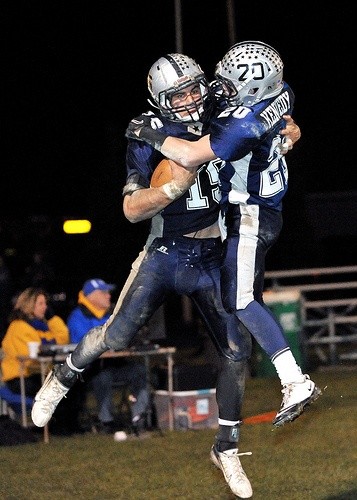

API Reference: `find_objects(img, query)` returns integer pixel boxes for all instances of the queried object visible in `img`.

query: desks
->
[16,347,176,444]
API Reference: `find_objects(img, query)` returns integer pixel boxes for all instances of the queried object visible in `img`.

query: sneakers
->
[31,364,70,427]
[273,374,323,428]
[210,444,253,498]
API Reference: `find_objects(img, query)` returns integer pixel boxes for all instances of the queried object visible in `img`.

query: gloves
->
[126,111,169,151]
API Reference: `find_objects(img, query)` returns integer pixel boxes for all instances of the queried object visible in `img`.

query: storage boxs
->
[154,388,219,432]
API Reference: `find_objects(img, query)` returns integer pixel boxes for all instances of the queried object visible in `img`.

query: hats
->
[83,279,115,297]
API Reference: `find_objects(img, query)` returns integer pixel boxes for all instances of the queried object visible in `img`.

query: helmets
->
[209,41,284,107]
[147,54,209,123]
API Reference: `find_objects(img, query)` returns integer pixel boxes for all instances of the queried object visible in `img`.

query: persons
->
[31,53,301,499]
[125,40,323,430]
[1,277,156,434]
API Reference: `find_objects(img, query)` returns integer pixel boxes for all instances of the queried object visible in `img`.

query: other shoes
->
[103,420,143,436]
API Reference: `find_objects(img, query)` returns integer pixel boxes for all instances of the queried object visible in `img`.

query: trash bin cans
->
[247,290,304,379]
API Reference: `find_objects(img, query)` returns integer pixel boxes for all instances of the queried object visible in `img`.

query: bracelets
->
[162,181,182,199]
